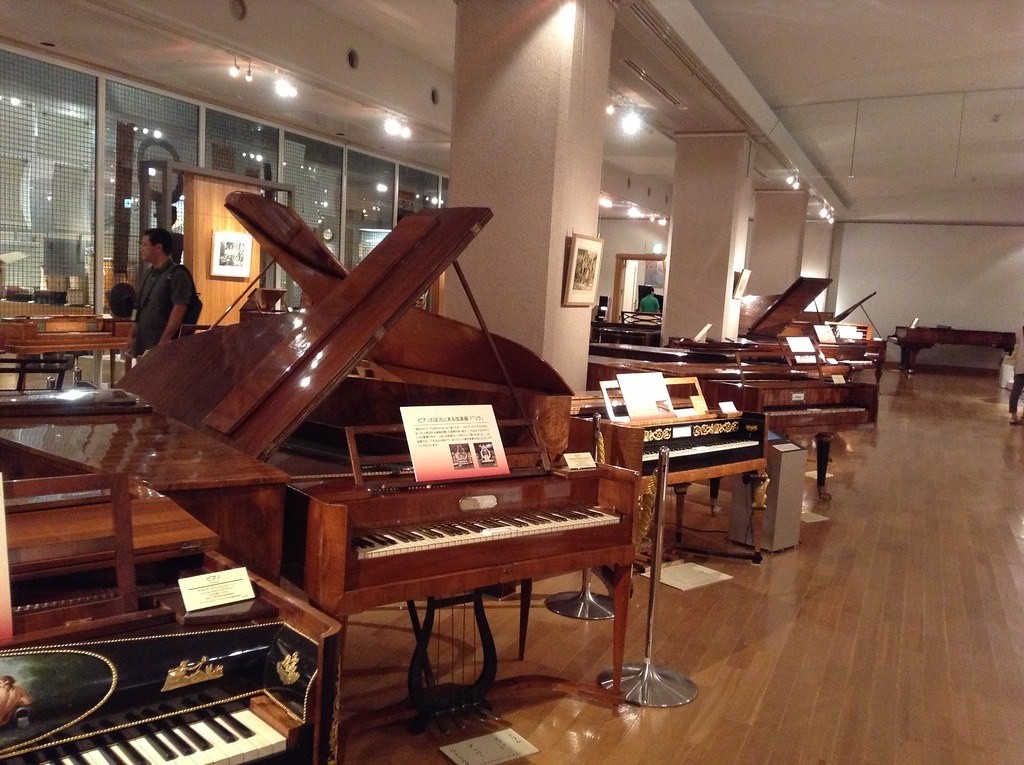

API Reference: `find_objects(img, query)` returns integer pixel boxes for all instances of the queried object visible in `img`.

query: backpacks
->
[170,265,203,339]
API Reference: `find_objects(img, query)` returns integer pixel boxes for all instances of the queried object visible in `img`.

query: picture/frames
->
[733,269,752,301]
[562,233,603,307]
[209,229,252,278]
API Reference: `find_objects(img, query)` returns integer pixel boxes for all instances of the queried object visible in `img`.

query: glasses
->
[141,242,152,247]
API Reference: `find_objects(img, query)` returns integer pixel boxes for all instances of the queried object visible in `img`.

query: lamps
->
[229,58,241,78]
[245,63,253,82]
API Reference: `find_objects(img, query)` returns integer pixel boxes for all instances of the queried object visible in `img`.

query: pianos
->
[746,276,889,383]
[698,350,880,518]
[1,469,346,765]
[565,370,771,566]
[888,316,1016,375]
[108,185,649,739]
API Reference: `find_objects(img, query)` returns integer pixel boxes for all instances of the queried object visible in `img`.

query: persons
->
[639,287,660,313]
[1008,325,1024,424]
[125,228,194,361]
